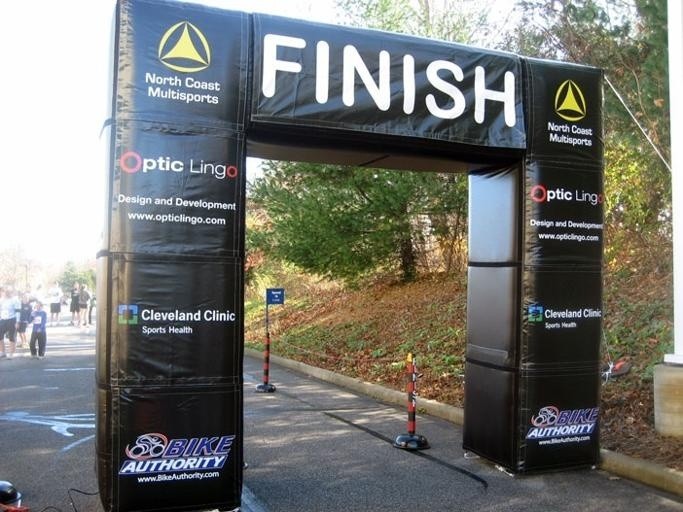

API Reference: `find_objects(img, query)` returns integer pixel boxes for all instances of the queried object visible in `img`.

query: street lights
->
[20,264,28,290]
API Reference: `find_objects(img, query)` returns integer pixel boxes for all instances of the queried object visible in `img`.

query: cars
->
[61,296,71,304]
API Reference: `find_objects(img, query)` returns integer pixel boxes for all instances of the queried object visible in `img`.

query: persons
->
[0,278,91,360]
